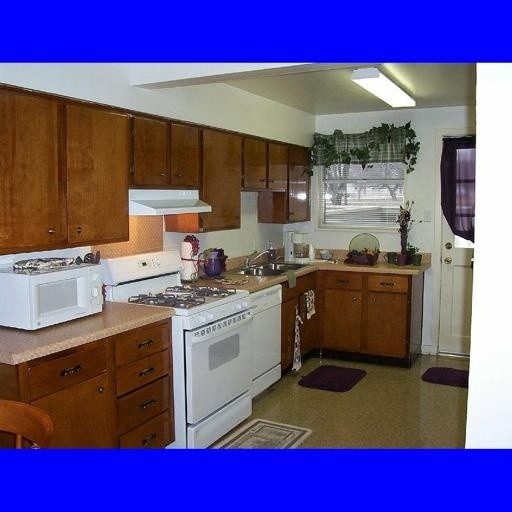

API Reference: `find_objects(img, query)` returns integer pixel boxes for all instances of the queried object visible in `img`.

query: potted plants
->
[343,246,381,266]
[395,199,422,267]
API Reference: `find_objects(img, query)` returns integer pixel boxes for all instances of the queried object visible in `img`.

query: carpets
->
[421,367,468,389]
[299,365,366,393]
[212,419,314,450]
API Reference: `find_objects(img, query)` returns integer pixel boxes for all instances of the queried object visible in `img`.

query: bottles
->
[267,239,277,262]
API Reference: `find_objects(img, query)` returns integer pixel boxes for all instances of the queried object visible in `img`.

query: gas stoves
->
[127,283,251,329]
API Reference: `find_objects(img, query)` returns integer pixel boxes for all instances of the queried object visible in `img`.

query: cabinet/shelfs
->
[165,129,240,233]
[241,137,287,191]
[256,145,310,222]
[116,321,175,450]
[0,337,117,450]
[319,270,424,363]
[0,85,129,254]
[282,271,318,371]
[130,115,200,191]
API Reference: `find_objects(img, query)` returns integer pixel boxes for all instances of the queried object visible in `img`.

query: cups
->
[394,253,407,266]
[409,254,423,266]
[383,251,398,264]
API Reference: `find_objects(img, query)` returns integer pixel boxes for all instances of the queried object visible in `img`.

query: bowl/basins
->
[320,249,334,259]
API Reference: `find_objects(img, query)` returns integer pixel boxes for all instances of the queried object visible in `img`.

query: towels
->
[291,305,303,371]
[303,290,317,321]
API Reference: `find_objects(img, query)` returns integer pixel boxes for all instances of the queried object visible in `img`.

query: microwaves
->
[0,263,104,332]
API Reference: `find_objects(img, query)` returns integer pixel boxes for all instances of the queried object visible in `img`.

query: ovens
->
[174,307,253,448]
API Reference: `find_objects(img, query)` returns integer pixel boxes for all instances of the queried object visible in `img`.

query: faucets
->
[243,250,270,267]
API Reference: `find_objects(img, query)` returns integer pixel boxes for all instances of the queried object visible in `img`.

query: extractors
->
[127,188,212,216]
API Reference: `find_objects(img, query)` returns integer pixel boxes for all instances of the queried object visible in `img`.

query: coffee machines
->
[283,230,316,261]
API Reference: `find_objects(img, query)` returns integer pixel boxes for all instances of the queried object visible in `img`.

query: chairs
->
[0,399,54,449]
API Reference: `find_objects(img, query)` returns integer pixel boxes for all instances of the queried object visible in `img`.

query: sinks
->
[234,262,305,277]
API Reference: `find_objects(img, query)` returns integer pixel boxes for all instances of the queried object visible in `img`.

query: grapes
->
[344,250,357,264]
[208,248,228,272]
[185,235,199,255]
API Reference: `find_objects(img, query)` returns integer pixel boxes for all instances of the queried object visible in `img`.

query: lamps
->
[351,68,417,108]
